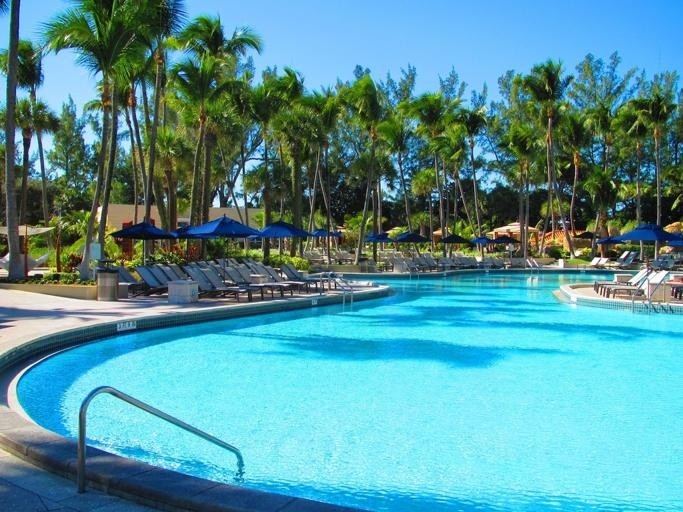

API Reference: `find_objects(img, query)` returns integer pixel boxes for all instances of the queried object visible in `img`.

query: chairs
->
[594,267,670,302]
[378,249,509,274]
[649,252,683,270]
[587,249,638,268]
[136,256,340,304]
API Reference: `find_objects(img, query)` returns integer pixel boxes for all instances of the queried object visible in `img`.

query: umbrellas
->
[170,222,222,265]
[437,234,471,256]
[309,227,341,255]
[362,232,395,260]
[183,213,259,263]
[245,218,314,258]
[469,235,497,258]
[392,230,430,257]
[107,216,178,267]
[570,222,683,269]
[491,234,521,251]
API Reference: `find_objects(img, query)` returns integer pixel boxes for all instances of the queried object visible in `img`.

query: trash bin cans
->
[95,258,120,301]
